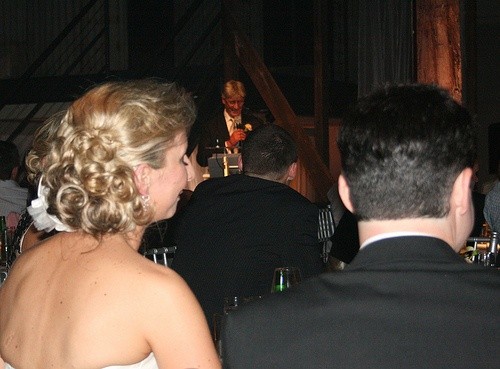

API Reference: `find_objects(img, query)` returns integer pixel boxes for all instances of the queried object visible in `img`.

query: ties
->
[229,119,237,137]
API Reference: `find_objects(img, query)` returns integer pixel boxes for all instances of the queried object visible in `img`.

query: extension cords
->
[223,155,229,177]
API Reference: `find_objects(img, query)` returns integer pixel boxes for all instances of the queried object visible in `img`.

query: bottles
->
[486,232,500,268]
[0,216,11,267]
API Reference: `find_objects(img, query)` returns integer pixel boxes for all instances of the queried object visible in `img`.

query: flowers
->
[243,123,253,132]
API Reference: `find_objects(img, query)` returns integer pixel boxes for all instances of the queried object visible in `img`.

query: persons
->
[172,124,320,343]
[196,78,265,167]
[0,78,222,369]
[220,82,500,369]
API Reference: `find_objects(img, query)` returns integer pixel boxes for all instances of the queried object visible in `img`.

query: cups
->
[223,296,240,316]
[270,266,303,295]
[2,225,15,268]
